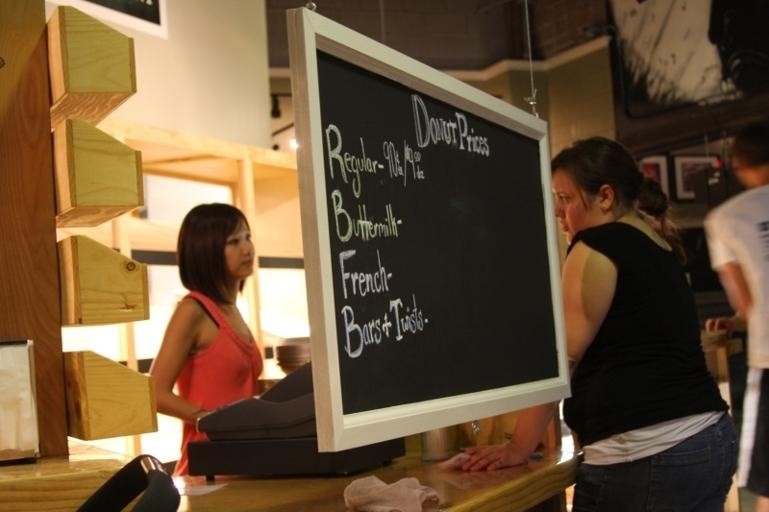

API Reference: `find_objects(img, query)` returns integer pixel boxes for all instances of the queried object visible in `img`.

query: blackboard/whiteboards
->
[285,7,574,455]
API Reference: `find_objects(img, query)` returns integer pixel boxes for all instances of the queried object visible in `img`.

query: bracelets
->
[185,409,209,422]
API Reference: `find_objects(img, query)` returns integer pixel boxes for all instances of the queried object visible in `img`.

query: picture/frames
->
[672,154,721,203]
[639,153,673,203]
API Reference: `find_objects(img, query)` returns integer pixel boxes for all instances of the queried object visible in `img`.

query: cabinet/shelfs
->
[46,5,159,441]
[109,139,311,459]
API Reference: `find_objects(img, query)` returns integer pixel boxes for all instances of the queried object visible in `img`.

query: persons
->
[457,134,742,510]
[702,122,768,511]
[147,200,273,483]
